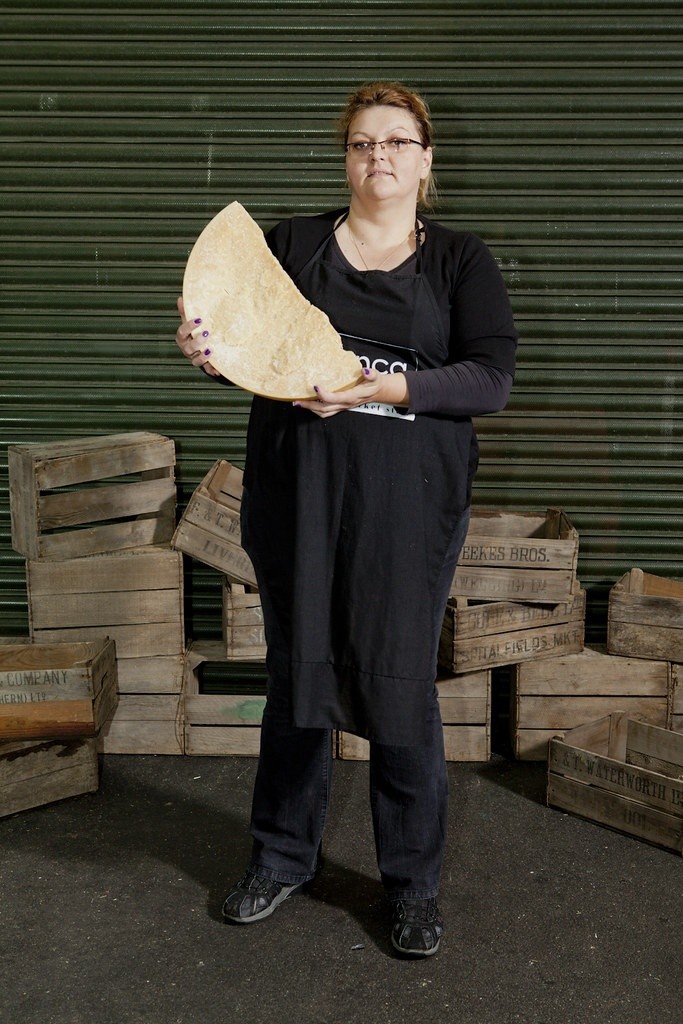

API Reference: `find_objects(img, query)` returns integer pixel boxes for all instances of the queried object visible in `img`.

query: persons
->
[175,79,521,957]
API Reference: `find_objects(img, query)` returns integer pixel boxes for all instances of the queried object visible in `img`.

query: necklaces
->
[348,219,414,269]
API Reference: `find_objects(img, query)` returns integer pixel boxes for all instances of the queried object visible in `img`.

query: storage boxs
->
[1,429,682,854]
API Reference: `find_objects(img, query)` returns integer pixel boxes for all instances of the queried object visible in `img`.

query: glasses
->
[345,137,425,157]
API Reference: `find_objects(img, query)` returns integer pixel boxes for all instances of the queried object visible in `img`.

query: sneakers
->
[391,892,444,954]
[222,871,303,923]
